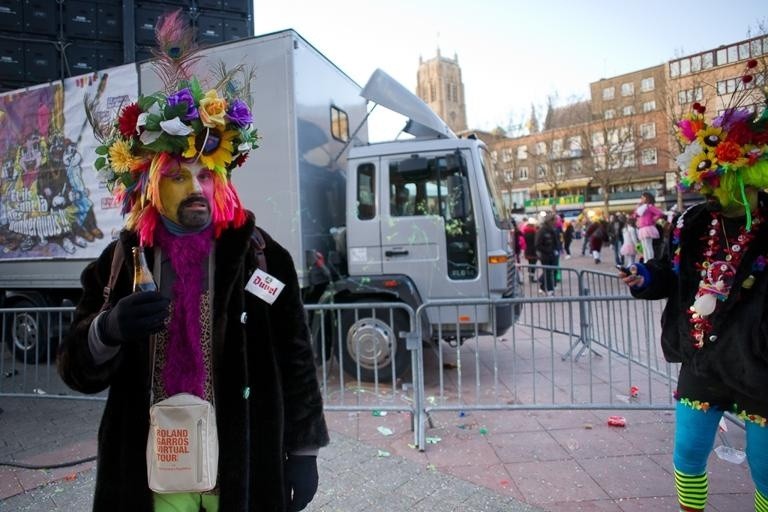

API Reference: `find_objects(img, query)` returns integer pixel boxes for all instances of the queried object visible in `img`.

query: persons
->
[617,176,768,512]
[54,160,330,512]
[514,190,677,297]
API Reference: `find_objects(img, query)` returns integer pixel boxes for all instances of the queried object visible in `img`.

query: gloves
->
[284,453,319,512]
[95,289,171,345]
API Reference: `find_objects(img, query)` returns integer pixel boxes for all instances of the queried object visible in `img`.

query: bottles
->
[132,247,164,335]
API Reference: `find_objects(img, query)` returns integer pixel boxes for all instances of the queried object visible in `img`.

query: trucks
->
[0,29,524,384]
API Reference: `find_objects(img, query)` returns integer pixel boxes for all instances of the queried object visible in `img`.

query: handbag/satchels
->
[144,390,219,493]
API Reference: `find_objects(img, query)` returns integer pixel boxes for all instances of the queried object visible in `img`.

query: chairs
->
[395,186,410,216]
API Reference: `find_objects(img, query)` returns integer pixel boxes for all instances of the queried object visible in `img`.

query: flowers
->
[92,73,262,191]
[675,58,767,190]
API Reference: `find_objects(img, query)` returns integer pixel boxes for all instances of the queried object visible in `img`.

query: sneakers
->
[537,288,555,297]
